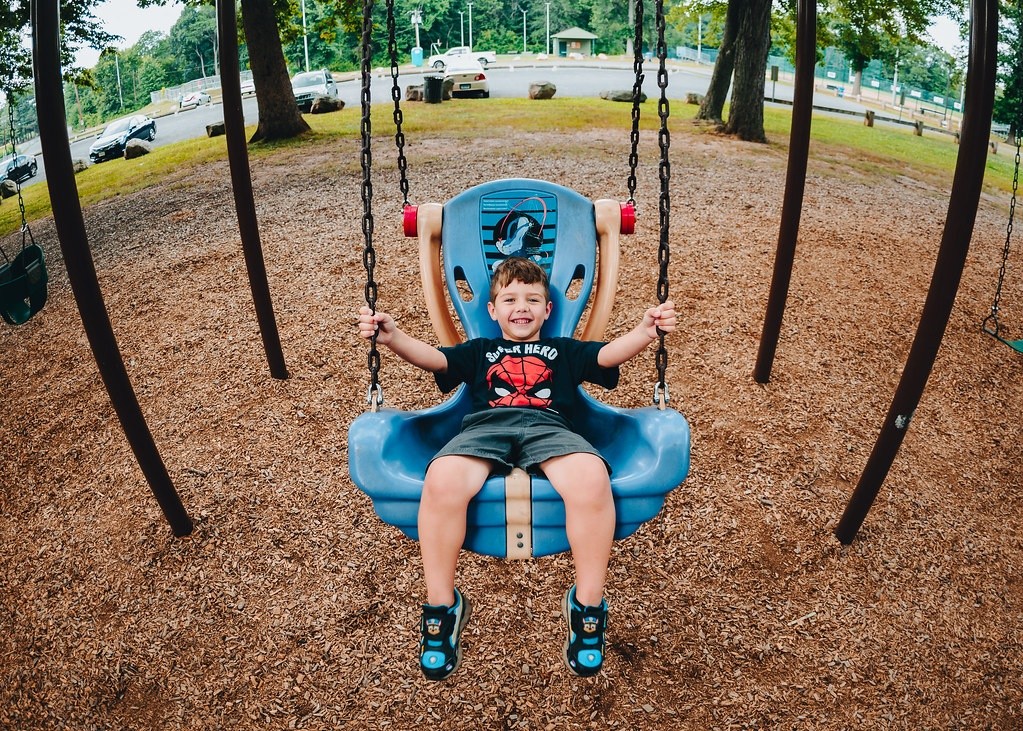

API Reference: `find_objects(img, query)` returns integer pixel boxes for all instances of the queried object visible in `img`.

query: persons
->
[358,257,676,682]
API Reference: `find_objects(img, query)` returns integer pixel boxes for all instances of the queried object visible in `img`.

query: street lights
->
[544,3,551,55]
[457,11,466,47]
[521,10,529,54]
[467,2,474,52]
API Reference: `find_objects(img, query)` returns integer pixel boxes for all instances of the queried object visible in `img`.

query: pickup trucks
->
[428,46,496,70]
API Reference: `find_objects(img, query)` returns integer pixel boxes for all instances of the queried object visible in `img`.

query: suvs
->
[290,68,338,114]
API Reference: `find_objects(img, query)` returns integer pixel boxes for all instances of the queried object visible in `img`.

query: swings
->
[981,77,1023,356]
[1,0,51,326]
[348,2,692,562]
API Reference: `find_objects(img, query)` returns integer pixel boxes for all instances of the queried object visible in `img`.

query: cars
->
[240,79,256,96]
[89,115,157,164]
[0,155,38,195]
[443,60,489,99]
[182,92,211,107]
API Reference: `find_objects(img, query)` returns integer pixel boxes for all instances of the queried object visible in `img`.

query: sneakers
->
[419,586,472,682]
[560,584,607,678]
